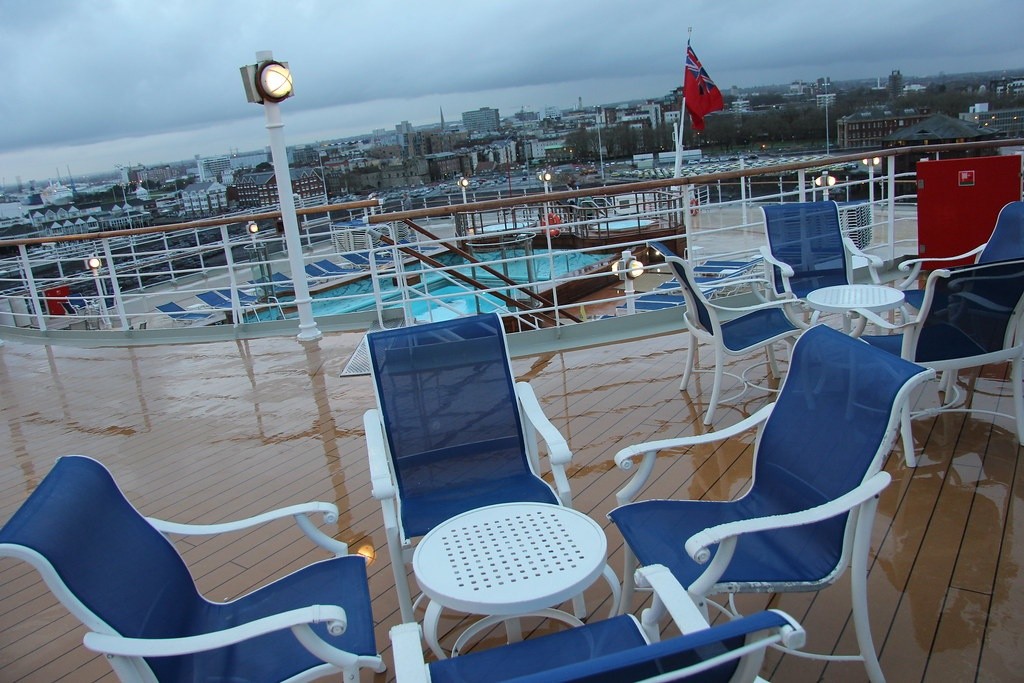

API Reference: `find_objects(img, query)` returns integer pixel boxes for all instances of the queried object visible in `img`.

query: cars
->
[610,163,740,179]
[688,154,757,164]
[378,187,432,198]
[440,176,506,193]
[745,156,856,176]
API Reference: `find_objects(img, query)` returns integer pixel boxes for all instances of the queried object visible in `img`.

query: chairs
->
[388,564,808,683]
[847,256,1024,467]
[646,240,805,424]
[893,201,1024,391]
[0,455,387,683]
[58,287,123,330]
[612,322,935,683]
[154,273,318,328]
[782,199,872,257]
[304,240,439,283]
[593,257,764,322]
[759,200,884,367]
[362,312,586,623]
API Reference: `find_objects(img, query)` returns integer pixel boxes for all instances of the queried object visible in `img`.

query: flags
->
[683,41,723,130]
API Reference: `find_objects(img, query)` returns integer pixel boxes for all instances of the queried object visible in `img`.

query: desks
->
[807,284,910,327]
[413,501,623,662]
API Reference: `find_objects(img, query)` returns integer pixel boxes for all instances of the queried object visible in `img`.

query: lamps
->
[256,60,293,103]
[612,262,619,276]
[460,179,469,187]
[626,259,643,278]
[88,258,100,268]
[544,173,551,181]
[249,223,259,233]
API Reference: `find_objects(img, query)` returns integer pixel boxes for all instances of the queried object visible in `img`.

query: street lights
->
[863,156,880,223]
[539,170,552,213]
[815,171,836,201]
[240,52,323,343]
[611,250,644,315]
[245,220,270,303]
[457,177,470,229]
[84,254,112,329]
